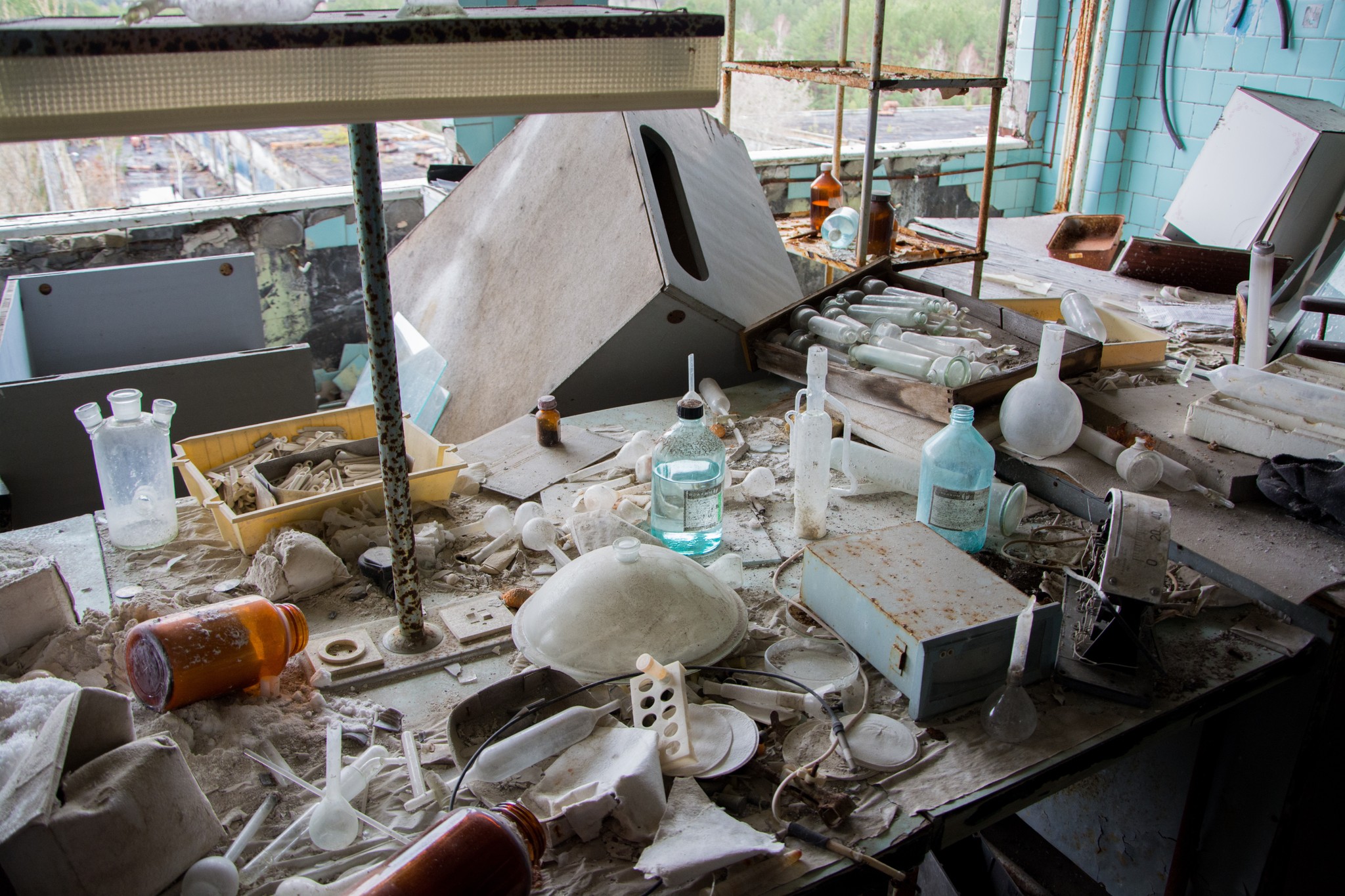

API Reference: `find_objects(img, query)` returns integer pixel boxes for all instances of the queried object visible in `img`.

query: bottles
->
[75,387,177,550]
[811,160,844,230]
[867,189,897,258]
[821,206,859,248]
[916,404,995,554]
[536,396,562,446]
[998,323,1083,456]
[650,397,725,555]
[126,594,309,713]
[1060,290,1108,344]
[348,801,546,896]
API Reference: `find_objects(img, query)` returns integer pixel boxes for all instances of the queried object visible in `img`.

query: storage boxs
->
[172,402,469,555]
[743,255,1103,424]
[1047,215,1125,271]
[982,296,1169,366]
[800,522,1062,720]
[1184,353,1345,460]
[250,437,415,510]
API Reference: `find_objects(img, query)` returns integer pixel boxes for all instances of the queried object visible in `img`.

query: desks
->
[1,212,1345,896]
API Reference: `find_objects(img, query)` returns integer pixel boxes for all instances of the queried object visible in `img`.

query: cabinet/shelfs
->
[1152,86,1345,304]
[386,109,803,444]
[724,1,1012,298]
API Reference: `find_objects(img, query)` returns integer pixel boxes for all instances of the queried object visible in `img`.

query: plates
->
[785,591,837,639]
[764,637,861,693]
